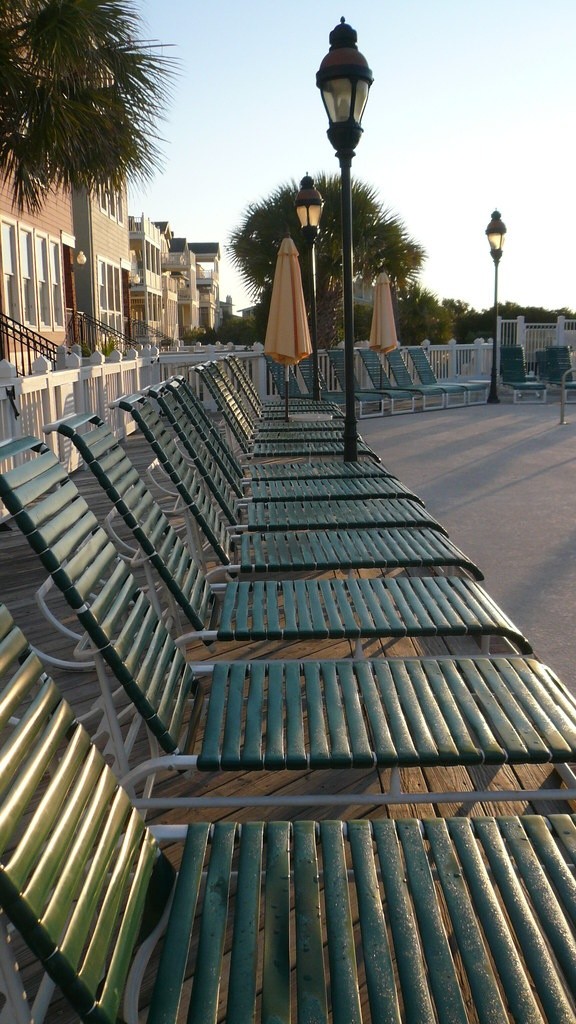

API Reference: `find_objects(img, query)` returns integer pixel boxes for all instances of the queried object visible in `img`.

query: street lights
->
[484,208,507,403]
[296,170,328,402]
[315,18,372,464]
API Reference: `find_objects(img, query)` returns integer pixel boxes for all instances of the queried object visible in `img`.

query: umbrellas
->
[368,273,397,410]
[265,230,313,421]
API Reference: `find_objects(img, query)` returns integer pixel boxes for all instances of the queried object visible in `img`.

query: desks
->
[288,413,332,421]
[468,379,491,384]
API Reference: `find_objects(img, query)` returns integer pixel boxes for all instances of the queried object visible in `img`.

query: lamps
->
[76,251,87,267]
[128,274,140,288]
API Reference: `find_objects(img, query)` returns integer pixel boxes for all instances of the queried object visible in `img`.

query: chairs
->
[0,345,576,1024]
